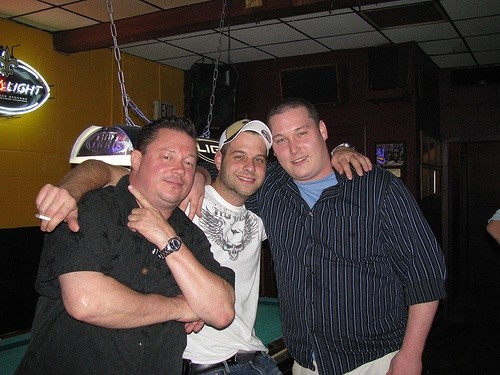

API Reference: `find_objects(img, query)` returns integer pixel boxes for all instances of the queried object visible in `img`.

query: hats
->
[219,119,273,156]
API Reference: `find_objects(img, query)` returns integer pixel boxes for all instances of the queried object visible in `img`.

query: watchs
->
[156,236,182,259]
[331,142,355,156]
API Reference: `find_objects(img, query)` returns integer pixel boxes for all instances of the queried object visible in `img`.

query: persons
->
[14,116,235,375]
[179,99,447,374]
[36,117,372,375]
[486,209,500,242]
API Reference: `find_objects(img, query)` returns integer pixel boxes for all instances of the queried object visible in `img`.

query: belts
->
[191,351,261,374]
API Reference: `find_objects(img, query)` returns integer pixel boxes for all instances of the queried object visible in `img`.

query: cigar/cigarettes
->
[35,213,52,221]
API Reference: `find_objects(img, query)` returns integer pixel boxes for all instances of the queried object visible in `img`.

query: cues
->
[271,348,289,360]
